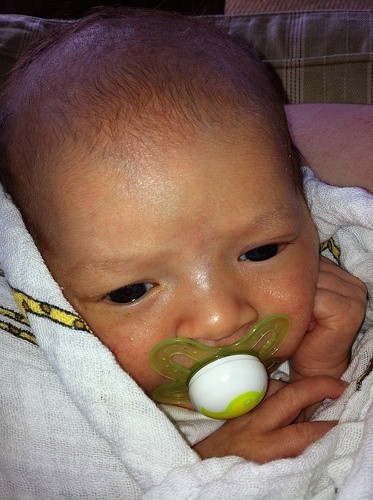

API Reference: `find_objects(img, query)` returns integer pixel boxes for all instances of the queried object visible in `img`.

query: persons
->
[1,10,373,500]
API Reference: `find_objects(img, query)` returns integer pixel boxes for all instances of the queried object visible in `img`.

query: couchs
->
[0,10,373,195]
